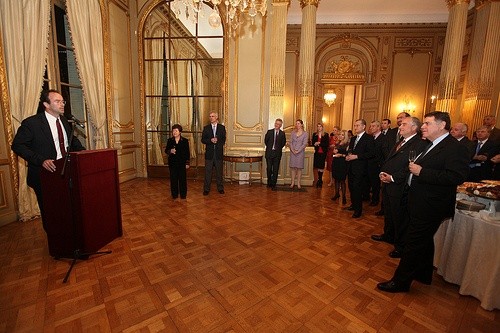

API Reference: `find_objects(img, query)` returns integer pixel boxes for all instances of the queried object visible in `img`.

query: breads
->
[456,180,500,212]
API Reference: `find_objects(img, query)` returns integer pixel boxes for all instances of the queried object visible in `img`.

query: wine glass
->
[408,150,416,174]
[488,200,496,217]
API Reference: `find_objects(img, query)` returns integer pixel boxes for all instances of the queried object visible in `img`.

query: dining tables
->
[432,186,500,312]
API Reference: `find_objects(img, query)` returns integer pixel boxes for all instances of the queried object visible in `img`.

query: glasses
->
[53,100,66,105]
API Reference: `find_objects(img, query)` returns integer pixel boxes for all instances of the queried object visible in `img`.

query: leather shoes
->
[389,248,402,258]
[375,210,384,217]
[411,274,433,284]
[344,205,363,218]
[362,196,380,206]
[377,279,410,294]
[371,234,392,245]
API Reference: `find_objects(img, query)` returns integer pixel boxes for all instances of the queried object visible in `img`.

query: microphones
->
[68,114,85,129]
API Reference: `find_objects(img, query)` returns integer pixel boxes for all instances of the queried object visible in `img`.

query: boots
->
[316,172,324,187]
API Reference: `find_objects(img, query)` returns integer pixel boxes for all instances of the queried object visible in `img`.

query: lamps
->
[324,89,337,107]
[172,0,268,38]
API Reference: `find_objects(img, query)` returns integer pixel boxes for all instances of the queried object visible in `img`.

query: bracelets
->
[342,154,343,157]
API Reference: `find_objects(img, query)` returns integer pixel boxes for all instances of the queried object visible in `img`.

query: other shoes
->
[275,131,278,147]
[171,194,186,200]
[203,190,210,196]
[219,190,225,194]
[267,182,276,188]
[213,126,216,139]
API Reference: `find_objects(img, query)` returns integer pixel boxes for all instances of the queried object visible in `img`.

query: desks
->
[223,155,264,186]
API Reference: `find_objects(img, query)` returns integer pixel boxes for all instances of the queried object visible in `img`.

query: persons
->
[289,119,307,191]
[10,89,87,256]
[450,115,500,185]
[264,118,286,191]
[201,110,226,196]
[345,111,459,293]
[312,122,354,205]
[165,124,190,199]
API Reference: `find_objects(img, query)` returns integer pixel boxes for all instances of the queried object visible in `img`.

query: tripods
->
[54,122,112,283]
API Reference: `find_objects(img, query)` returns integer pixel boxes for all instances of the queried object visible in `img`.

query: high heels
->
[331,194,347,205]
[289,182,303,190]
[327,181,335,186]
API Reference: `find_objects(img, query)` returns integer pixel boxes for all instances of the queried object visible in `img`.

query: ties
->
[383,131,385,135]
[373,135,376,139]
[475,142,482,155]
[396,130,402,143]
[55,119,69,158]
[353,135,359,150]
[407,142,433,178]
[395,140,406,153]
